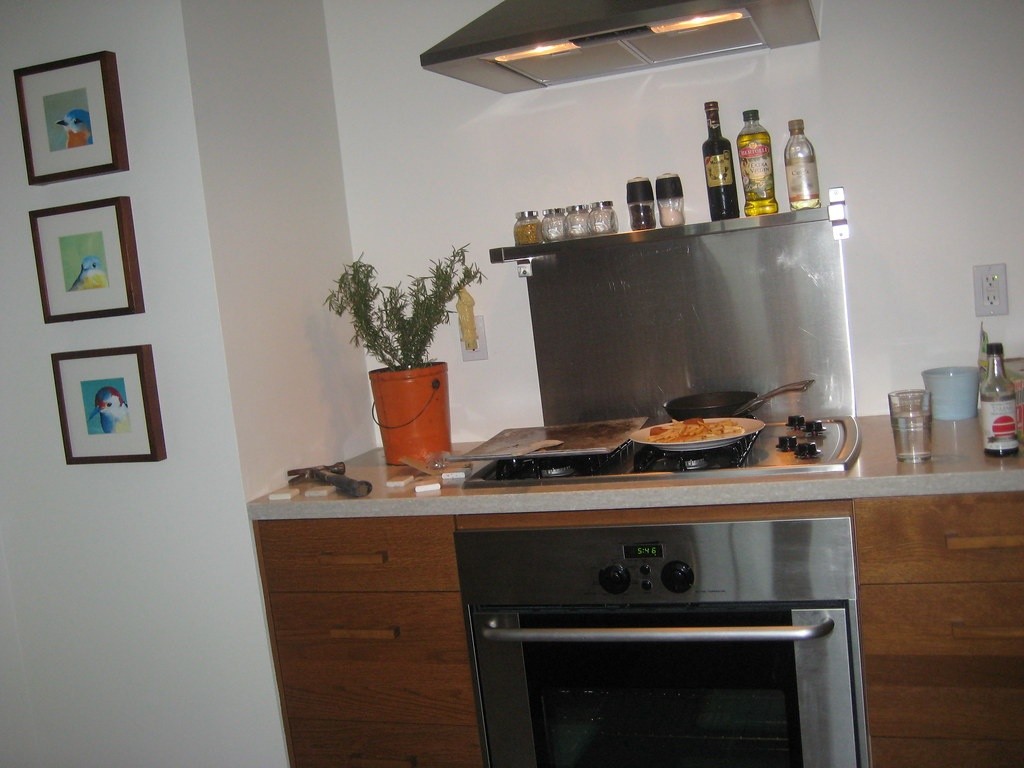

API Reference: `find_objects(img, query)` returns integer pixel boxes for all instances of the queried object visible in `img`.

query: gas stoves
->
[464,417,856,486]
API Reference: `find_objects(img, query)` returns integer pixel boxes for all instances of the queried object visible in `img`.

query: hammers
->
[287,461,373,497]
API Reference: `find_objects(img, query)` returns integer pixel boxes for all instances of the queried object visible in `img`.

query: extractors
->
[419,0,821,95]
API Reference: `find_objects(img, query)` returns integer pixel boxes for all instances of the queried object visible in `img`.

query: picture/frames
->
[51,344,167,464]
[28,196,146,323]
[13,51,131,186]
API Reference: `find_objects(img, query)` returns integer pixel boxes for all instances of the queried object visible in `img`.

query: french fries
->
[645,418,744,443]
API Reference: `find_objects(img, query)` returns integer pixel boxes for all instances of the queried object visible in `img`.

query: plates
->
[628,418,766,450]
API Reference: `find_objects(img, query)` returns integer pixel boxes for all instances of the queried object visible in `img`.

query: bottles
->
[512,199,619,246]
[655,173,685,228]
[626,178,657,231]
[980,342,1021,458]
[784,118,822,211]
[737,108,780,217]
[702,101,741,221]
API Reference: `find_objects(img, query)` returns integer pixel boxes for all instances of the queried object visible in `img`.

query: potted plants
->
[323,242,488,467]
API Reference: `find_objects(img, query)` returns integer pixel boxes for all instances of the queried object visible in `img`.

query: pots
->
[662,379,815,419]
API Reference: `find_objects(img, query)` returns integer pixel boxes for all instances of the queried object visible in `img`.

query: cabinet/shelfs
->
[253,515,488,768]
[854,490,1024,768]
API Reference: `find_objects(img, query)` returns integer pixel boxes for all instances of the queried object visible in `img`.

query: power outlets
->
[973,263,1009,317]
[459,315,488,362]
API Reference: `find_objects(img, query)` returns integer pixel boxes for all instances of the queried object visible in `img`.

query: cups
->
[888,389,932,465]
[921,367,979,421]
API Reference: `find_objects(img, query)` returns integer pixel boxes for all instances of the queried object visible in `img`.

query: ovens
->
[449,516,873,768]
